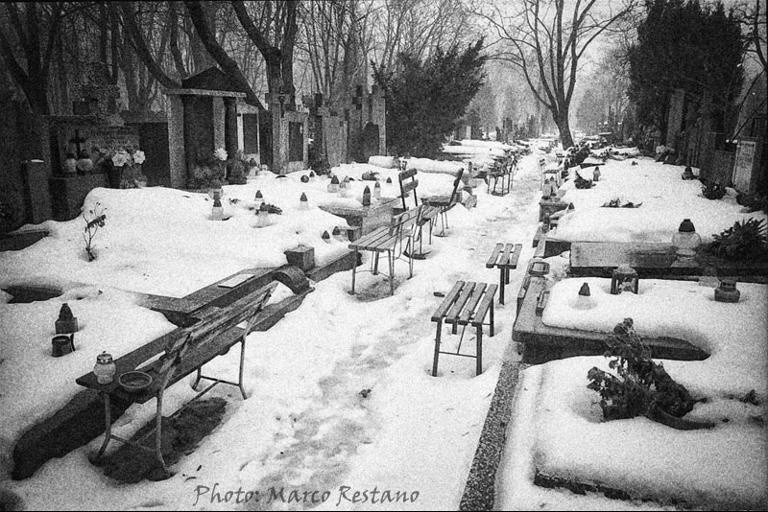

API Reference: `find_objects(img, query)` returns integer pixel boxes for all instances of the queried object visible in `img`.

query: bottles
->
[94,354,116,385]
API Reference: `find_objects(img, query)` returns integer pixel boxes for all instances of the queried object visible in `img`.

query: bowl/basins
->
[119,371,153,393]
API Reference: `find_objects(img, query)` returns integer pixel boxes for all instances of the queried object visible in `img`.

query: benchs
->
[78,156,569,481]
